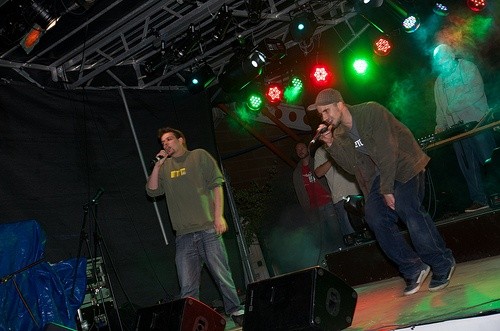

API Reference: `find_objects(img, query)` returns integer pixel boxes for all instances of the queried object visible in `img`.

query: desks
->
[424,121,500,150]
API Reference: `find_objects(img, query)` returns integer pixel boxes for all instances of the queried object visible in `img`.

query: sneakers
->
[403,266,431,296]
[464,203,489,213]
[428,258,456,293]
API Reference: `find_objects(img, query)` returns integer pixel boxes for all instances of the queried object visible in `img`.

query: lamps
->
[27,0,62,32]
[145,0,487,113]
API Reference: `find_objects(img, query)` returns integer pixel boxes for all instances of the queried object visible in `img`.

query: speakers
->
[242,265,358,331]
[132,297,226,331]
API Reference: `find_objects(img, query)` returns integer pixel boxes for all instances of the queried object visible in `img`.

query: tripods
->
[89,204,137,331]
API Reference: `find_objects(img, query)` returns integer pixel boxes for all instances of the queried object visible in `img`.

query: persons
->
[293,142,346,268]
[314,142,365,242]
[146,128,245,325]
[307,88,456,296]
[433,44,500,212]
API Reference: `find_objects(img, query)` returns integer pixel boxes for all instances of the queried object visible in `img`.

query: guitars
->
[418,115,479,148]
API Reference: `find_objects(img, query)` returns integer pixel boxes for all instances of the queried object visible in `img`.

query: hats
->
[307,88,344,111]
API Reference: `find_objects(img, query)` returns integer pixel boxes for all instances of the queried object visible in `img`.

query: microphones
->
[91,187,104,204]
[311,119,332,143]
[151,148,169,165]
